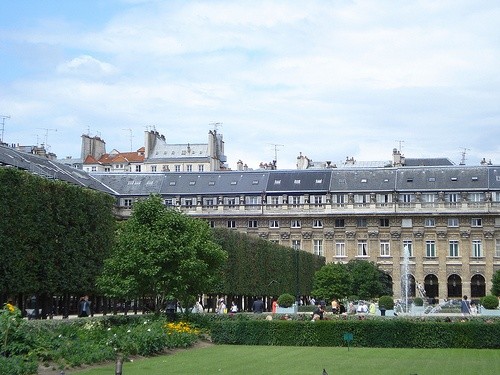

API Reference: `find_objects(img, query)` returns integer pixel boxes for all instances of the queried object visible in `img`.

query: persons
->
[369,300,375,314]
[320,298,326,311]
[310,301,323,322]
[192,302,203,312]
[461,295,472,315]
[300,299,303,306]
[310,297,316,306]
[79,295,91,317]
[331,298,337,314]
[348,302,356,315]
[218,301,226,314]
[358,302,368,313]
[339,302,345,314]
[432,298,436,308]
[230,300,239,313]
[252,296,265,314]
[271,299,279,314]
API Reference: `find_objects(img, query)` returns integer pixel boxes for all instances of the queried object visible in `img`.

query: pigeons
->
[322,368,328,375]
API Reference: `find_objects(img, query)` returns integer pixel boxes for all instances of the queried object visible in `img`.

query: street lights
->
[293,243,302,306]
